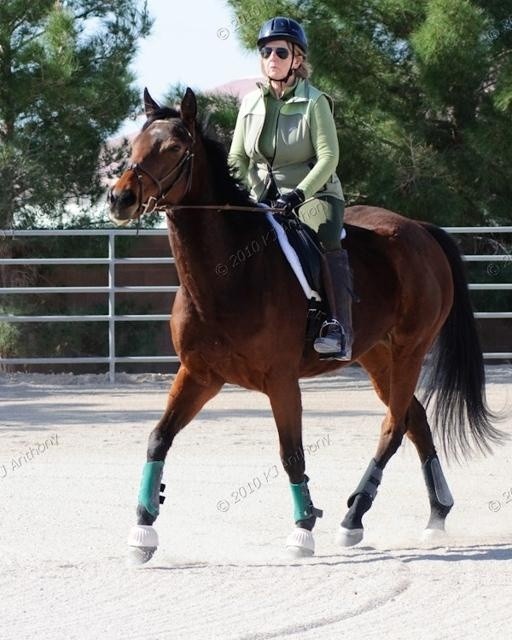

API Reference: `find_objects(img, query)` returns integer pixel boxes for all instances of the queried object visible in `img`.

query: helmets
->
[256,16,307,53]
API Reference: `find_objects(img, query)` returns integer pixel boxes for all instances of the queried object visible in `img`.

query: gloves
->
[274,189,305,215]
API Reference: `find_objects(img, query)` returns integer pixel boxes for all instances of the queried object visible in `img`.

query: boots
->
[314,250,354,362]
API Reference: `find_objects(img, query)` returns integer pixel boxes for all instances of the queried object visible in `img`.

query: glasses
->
[259,46,288,59]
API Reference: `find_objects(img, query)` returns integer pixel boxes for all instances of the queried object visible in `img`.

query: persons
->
[226,15,355,366]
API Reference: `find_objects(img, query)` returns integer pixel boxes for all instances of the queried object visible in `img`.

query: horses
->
[106,87,512,566]
